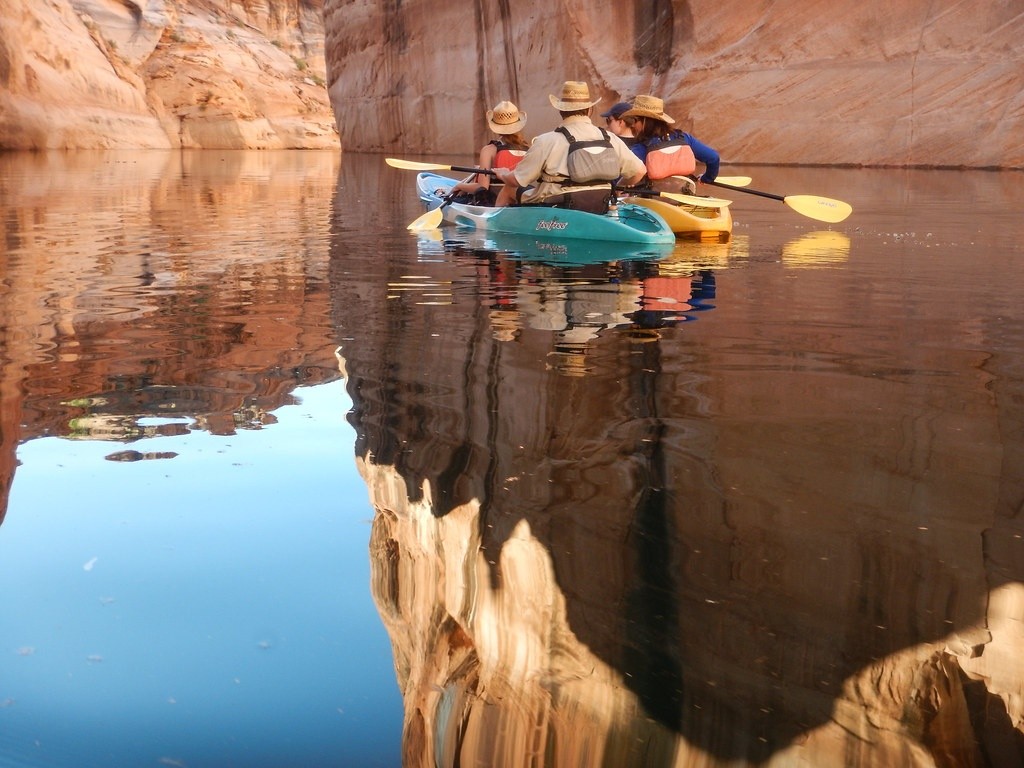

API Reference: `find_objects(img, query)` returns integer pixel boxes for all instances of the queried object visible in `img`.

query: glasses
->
[634,117,645,124]
[606,119,616,125]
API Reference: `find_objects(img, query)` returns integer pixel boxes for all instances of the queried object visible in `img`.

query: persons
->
[601,101,640,150]
[618,95,720,199]
[491,81,648,207]
[441,100,530,206]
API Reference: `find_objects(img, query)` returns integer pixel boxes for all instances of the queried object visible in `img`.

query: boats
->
[415,147,676,266]
[614,144,734,239]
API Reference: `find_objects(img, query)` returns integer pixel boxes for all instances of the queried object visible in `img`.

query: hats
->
[486,101,527,135]
[600,102,633,117]
[618,94,676,124]
[549,81,602,111]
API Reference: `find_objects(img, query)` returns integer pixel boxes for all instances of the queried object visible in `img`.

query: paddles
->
[691,174,853,224]
[387,155,733,211]
[710,176,752,188]
[405,164,480,228]
